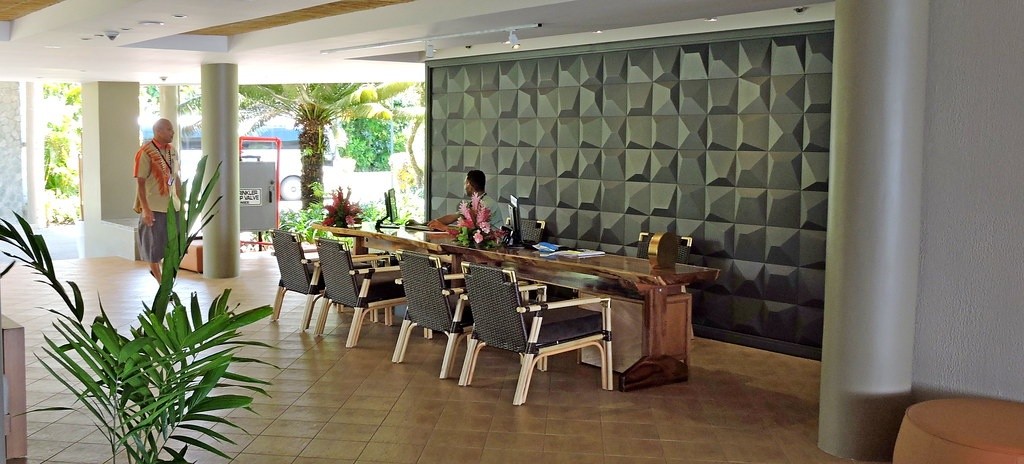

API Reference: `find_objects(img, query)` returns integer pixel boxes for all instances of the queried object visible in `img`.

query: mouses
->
[405,219,418,226]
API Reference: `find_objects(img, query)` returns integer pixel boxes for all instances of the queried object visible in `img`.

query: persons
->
[426,170,504,232]
[133,119,181,284]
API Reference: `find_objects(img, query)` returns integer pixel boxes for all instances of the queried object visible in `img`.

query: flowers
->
[321,185,365,229]
[456,191,506,250]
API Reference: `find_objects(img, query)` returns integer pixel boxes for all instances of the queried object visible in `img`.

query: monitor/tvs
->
[507,194,521,243]
[388,188,398,222]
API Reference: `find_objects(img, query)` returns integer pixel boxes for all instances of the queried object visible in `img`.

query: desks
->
[310,220,463,263]
[441,222,720,391]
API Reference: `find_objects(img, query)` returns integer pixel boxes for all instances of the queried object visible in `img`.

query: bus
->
[139,125,335,201]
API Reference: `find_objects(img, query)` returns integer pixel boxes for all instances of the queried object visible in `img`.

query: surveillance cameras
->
[106,31,119,40]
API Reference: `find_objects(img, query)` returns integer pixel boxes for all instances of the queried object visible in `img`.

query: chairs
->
[636,230,693,265]
[504,215,545,244]
[269,229,615,406]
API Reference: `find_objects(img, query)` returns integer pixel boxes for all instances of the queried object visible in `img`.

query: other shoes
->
[165,291,177,302]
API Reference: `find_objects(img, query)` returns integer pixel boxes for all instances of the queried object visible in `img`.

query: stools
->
[180,244,204,272]
[893,398,1024,464]
